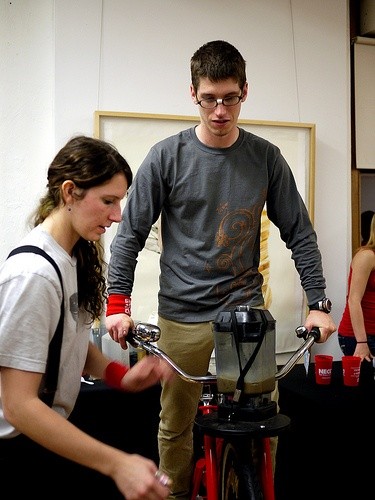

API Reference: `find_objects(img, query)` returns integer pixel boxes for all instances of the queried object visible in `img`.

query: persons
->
[106,41,336,500]
[0,136,174,500]
[338,215,375,362]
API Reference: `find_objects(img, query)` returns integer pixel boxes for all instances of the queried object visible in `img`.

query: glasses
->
[196,89,244,109]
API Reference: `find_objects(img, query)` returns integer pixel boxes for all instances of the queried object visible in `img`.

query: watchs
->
[308,298,332,314]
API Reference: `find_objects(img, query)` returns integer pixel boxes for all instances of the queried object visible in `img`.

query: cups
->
[341,356,362,386]
[313,354,333,384]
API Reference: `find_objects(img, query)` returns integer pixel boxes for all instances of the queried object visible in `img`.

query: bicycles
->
[128,322,320,500]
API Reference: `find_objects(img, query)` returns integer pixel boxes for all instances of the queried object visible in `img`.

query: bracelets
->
[358,342,367,343]
